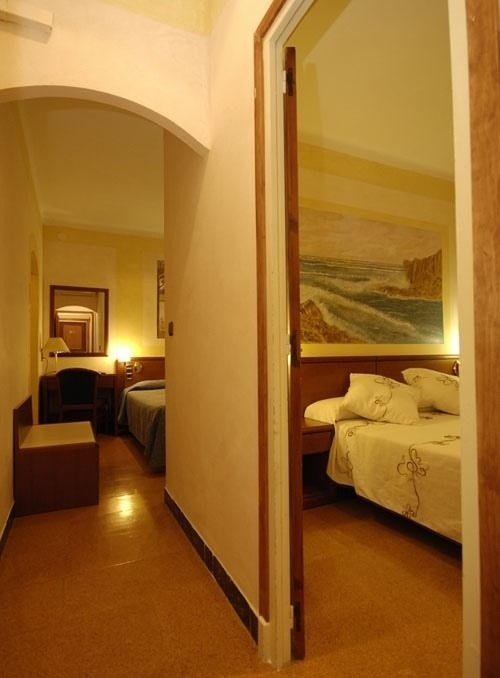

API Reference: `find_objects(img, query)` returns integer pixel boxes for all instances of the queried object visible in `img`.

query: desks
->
[40,373,115,434]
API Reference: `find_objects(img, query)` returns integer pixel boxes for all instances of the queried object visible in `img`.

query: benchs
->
[12,396,100,517]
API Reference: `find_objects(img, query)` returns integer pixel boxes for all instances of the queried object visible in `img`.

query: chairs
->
[55,368,109,439]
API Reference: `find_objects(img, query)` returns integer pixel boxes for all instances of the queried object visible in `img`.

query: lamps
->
[42,338,71,376]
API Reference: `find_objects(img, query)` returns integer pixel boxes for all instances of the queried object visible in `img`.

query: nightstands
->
[301,417,341,510]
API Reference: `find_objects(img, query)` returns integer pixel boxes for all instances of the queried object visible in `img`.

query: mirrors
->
[49,284,109,358]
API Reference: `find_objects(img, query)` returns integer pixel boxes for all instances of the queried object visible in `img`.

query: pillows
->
[340,367,460,425]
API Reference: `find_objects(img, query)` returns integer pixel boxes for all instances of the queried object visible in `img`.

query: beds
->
[301,355,461,545]
[114,356,165,471]
